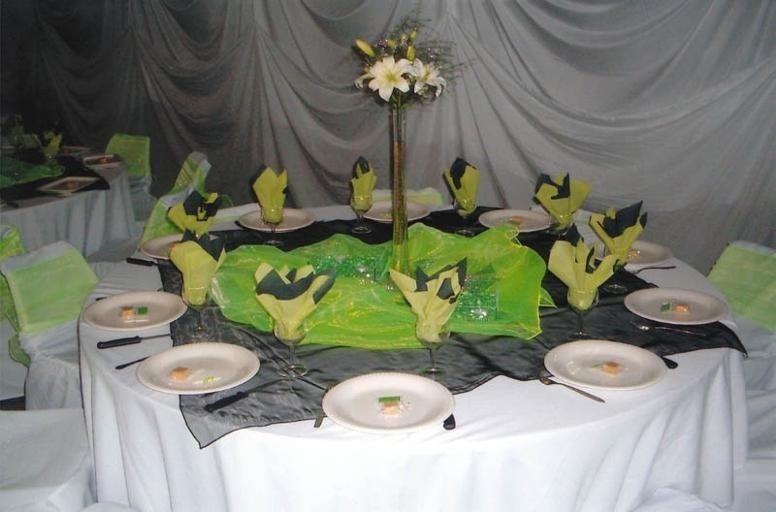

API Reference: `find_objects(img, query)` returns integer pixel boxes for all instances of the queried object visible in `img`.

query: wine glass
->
[257,203,285,246]
[179,277,216,339]
[413,316,451,376]
[348,192,376,236]
[452,194,477,234]
[601,248,632,295]
[271,318,312,379]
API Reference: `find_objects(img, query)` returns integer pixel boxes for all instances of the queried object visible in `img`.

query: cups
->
[546,210,573,239]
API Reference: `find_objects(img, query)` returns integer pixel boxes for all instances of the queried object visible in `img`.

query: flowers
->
[349,13,469,106]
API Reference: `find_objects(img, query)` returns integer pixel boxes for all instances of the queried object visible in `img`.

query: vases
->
[382,99,419,296]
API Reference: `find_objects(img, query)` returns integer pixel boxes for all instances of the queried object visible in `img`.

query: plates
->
[544,337,669,390]
[37,176,98,195]
[364,199,430,225]
[42,144,89,157]
[479,208,551,234]
[622,288,728,324]
[135,232,218,260]
[81,153,126,169]
[135,341,259,394]
[83,289,187,331]
[320,370,455,433]
[592,240,669,262]
[237,205,317,232]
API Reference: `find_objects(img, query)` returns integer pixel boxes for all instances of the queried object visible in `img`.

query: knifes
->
[121,256,171,270]
[205,377,282,411]
[95,323,214,351]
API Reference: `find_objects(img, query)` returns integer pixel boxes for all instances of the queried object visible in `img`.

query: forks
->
[313,384,335,428]
[620,265,676,277]
[627,317,709,340]
[535,372,605,403]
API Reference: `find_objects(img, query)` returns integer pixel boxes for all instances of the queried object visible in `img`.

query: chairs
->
[105,135,154,221]
[1,241,92,408]
[88,152,209,282]
[86,502,139,512]
[707,239,775,429]
[0,408,92,512]
